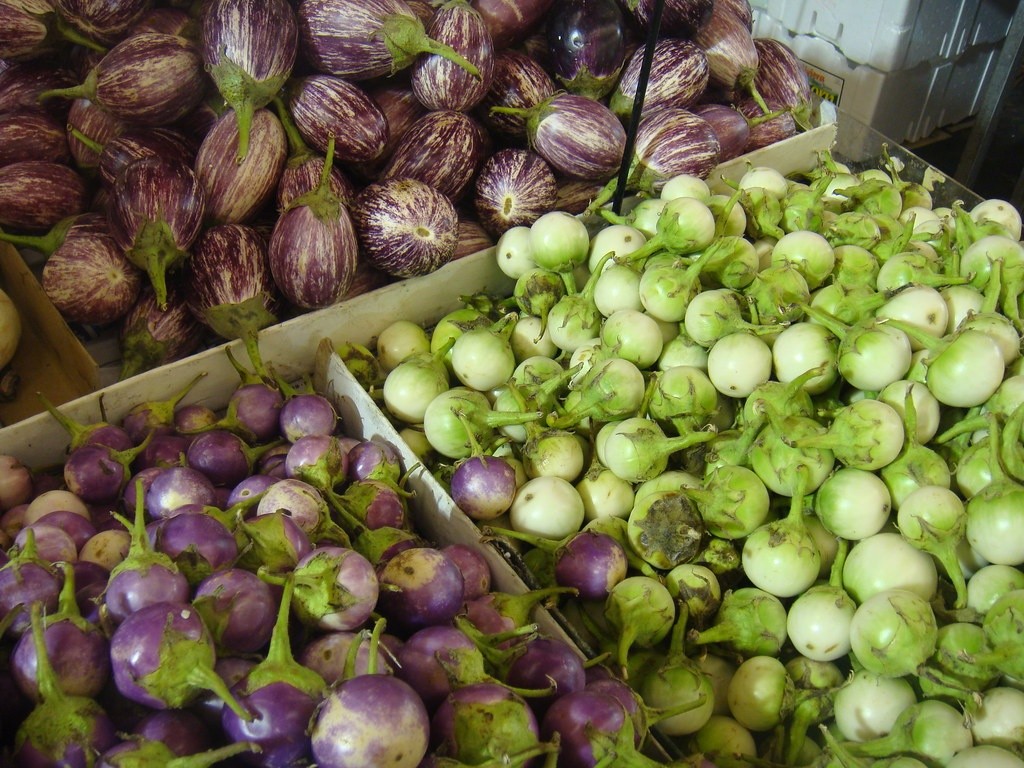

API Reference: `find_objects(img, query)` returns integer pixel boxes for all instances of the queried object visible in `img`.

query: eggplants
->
[0,1,1024,768]
